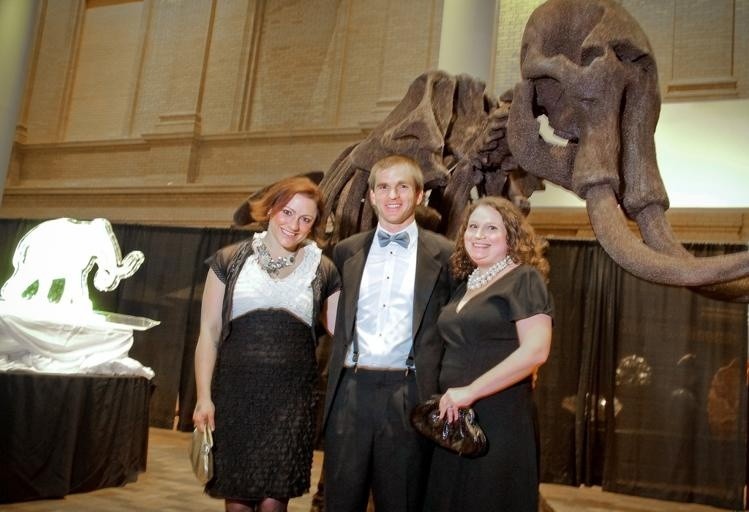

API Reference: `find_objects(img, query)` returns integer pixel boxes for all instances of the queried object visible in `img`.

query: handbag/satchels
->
[409,393,489,460]
[186,421,214,486]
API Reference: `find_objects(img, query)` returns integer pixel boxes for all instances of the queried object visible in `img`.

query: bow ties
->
[377,230,411,248]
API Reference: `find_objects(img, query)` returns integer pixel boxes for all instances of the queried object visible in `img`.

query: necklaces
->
[465,255,513,292]
[254,236,302,276]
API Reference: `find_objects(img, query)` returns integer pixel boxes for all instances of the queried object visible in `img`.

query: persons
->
[418,195,555,512]
[308,153,540,512]
[192,175,344,511]
